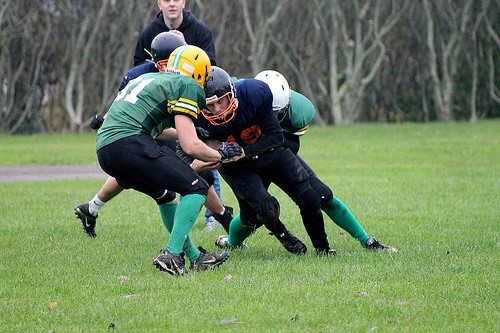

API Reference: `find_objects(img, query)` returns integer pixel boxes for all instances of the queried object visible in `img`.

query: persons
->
[210,68,398,254]
[186,64,336,254]
[133,0,224,231]
[92,42,229,275]
[75,31,246,251]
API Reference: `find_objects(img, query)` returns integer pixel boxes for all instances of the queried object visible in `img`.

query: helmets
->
[150,32,185,62]
[167,44,211,88]
[204,66,237,104]
[254,70,290,110]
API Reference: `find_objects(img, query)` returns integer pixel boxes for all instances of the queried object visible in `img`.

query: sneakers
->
[192,245,230,272]
[74,203,97,238]
[203,216,402,256]
[154,248,188,276]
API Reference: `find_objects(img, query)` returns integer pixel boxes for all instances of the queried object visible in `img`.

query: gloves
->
[88,114,104,131]
[217,142,243,159]
[195,126,210,138]
